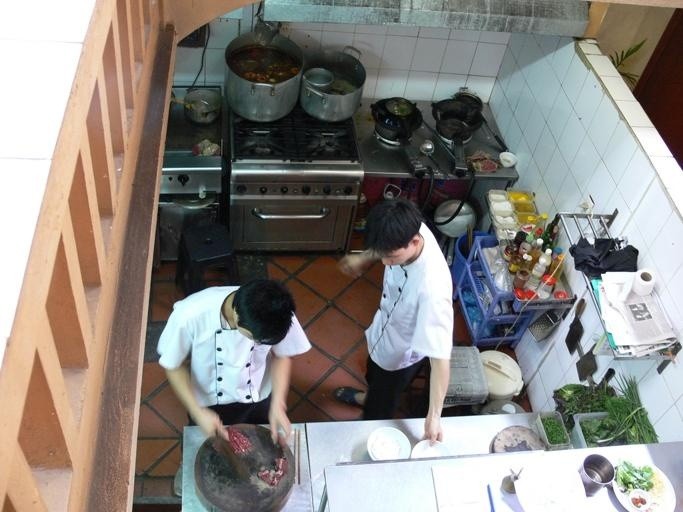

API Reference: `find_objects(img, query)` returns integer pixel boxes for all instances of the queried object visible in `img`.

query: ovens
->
[228,168,362,253]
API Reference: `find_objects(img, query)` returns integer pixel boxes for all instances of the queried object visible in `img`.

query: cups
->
[579,453,615,498]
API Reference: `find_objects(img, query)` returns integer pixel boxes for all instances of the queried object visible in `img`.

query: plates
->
[613,460,677,512]
[412,440,451,460]
[366,427,411,462]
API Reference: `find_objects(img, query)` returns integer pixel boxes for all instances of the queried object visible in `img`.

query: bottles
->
[503,213,571,313]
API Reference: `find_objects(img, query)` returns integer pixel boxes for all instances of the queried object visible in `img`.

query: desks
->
[305,409,574,511]
[182,423,314,511]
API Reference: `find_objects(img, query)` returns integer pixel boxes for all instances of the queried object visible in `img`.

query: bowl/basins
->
[303,67,347,96]
[497,151,517,169]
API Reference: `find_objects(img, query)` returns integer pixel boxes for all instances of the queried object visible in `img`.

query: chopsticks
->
[294,428,301,484]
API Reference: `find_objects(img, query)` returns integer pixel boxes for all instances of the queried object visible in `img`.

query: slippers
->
[332,386,365,408]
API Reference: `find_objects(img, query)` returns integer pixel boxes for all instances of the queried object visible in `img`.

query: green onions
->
[597,372,657,444]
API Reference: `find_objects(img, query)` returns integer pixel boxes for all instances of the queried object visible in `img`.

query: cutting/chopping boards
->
[491,425,548,452]
[195,424,294,511]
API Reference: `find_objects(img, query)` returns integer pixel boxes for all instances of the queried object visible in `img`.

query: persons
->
[332,195,455,448]
[155,273,314,502]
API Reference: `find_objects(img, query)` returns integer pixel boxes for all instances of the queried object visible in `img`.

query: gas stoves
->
[231,112,362,167]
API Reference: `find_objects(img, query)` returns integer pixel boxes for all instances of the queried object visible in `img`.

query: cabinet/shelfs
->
[455,234,537,353]
[349,176,473,256]
[319,440,682,510]
[558,206,682,375]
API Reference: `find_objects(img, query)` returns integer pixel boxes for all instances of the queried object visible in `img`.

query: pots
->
[224,21,305,123]
[430,98,484,141]
[369,94,423,142]
[171,89,221,124]
[301,44,368,123]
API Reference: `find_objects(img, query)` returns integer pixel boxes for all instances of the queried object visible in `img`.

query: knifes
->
[594,368,616,397]
[564,298,591,356]
[576,343,599,382]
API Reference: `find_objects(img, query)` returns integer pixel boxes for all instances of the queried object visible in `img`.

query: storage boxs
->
[536,410,570,448]
[571,412,613,448]
